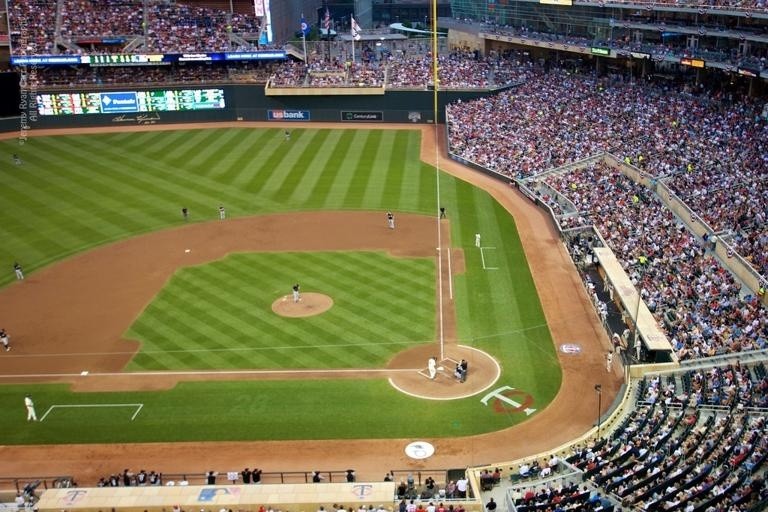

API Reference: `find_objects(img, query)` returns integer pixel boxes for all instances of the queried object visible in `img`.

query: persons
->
[386,207,396,229]
[219,203,225,220]
[24,393,38,421]
[182,205,189,220]
[428,353,437,381]
[0,327,12,351]
[14,261,24,282]
[11,151,20,165]
[292,281,301,300]
